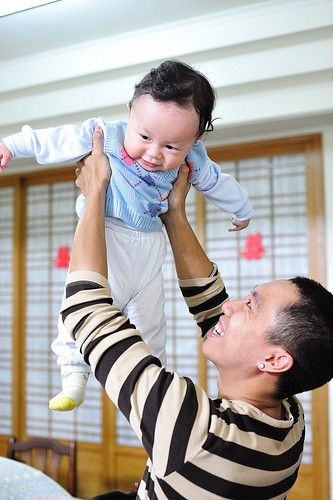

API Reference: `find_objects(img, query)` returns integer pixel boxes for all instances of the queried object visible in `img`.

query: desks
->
[0,456,78,500]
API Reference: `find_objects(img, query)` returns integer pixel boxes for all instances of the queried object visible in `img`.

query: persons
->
[0,60,254,411]
[59,128,333,500]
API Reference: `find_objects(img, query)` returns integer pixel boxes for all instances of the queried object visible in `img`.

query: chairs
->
[8,436,76,498]
